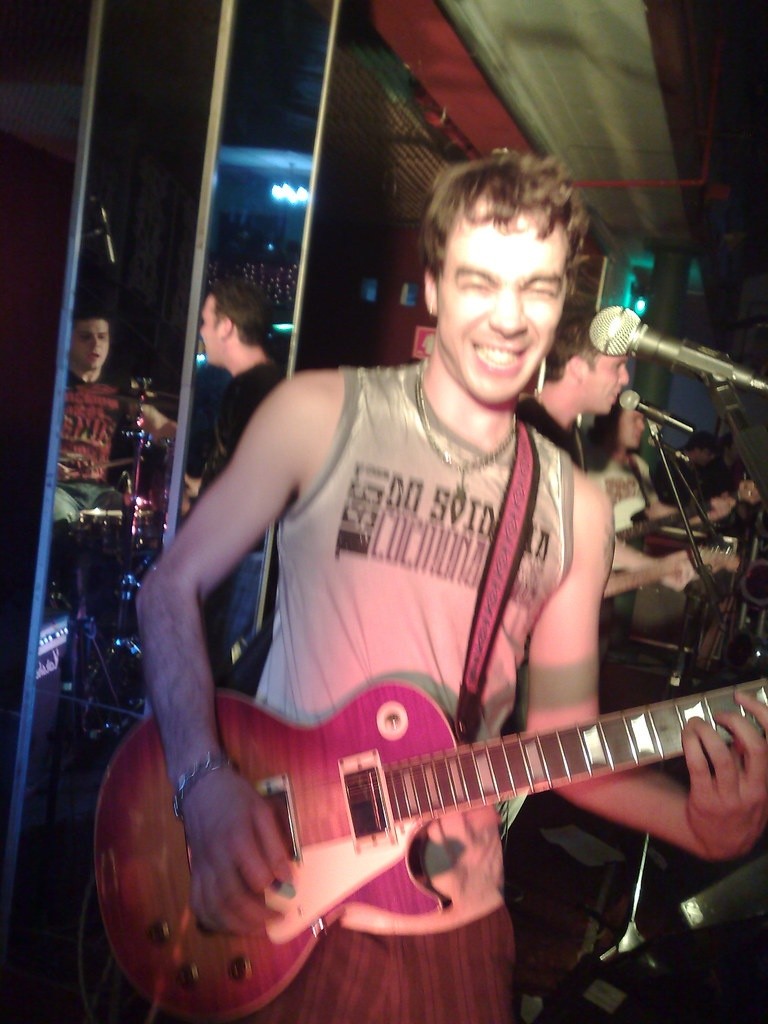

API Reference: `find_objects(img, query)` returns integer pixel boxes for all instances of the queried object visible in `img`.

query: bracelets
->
[170,750,236,819]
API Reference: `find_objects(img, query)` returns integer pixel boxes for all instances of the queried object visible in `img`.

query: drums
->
[77,505,163,638]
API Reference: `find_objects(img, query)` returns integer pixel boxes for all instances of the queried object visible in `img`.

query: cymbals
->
[84,385,181,408]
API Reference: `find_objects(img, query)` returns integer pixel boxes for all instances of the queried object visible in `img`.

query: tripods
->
[58,555,120,729]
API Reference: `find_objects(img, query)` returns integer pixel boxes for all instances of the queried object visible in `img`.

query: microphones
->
[99,208,116,264]
[589,306,768,395]
[619,389,694,434]
[647,435,689,463]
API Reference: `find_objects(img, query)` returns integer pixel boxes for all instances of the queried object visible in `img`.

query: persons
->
[54,279,177,551]
[134,150,768,1023]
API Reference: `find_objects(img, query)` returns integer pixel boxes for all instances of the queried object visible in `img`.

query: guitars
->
[94,679,768,1021]
[600,487,744,599]
